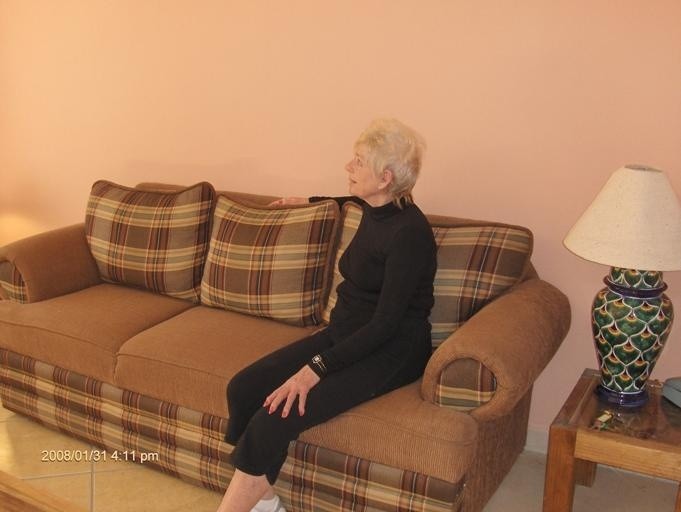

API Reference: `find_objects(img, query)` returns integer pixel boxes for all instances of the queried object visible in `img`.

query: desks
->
[542,368,681,511]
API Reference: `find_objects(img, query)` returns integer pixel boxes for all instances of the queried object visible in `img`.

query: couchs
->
[0,182,571,512]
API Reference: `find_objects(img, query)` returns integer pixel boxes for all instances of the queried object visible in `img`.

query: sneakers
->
[249,495,286,512]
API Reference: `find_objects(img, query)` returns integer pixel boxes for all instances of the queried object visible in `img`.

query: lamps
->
[560,163,680,410]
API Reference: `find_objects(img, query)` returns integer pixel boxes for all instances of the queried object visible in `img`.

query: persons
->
[216,116,440,511]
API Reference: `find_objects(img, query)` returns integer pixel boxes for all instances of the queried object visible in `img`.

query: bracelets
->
[312,355,328,373]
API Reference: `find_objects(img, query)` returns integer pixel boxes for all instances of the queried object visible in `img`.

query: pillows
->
[85,181,216,305]
[321,202,533,350]
[200,194,340,329]
